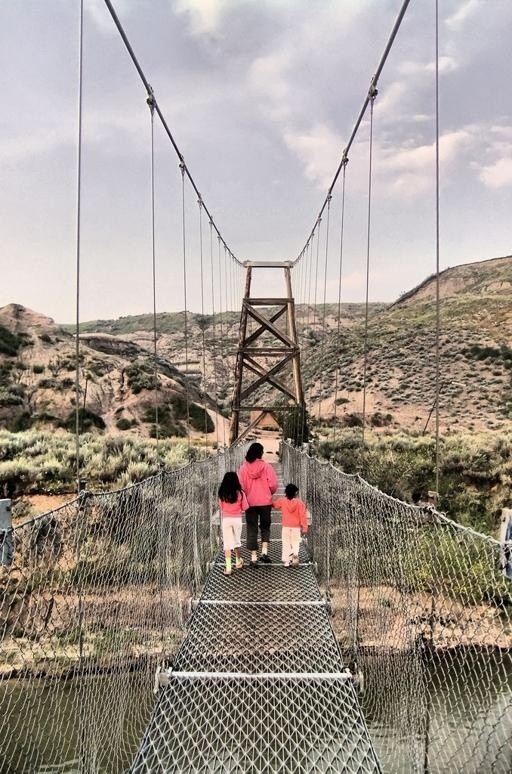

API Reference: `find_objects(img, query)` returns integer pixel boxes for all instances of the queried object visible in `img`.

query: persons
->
[217,471,249,575]
[238,442,279,566]
[272,483,308,568]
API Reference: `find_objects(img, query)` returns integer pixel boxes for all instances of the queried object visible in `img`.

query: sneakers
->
[223,553,300,576]
